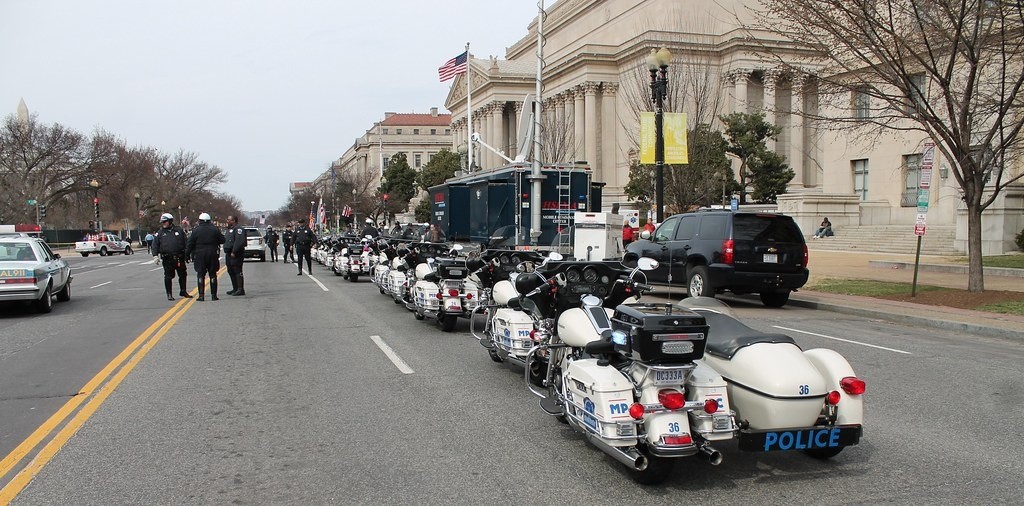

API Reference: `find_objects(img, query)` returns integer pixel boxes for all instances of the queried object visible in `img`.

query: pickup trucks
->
[74,234,131,256]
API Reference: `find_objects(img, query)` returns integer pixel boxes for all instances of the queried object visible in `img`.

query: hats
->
[407,223,412,226]
[298,219,304,223]
[286,224,292,228]
[267,224,273,229]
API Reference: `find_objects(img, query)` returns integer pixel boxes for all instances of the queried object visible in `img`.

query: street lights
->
[352,188,358,229]
[381,176,388,226]
[161,200,166,214]
[645,43,673,224]
[134,193,143,247]
[178,205,182,227]
[90,180,99,233]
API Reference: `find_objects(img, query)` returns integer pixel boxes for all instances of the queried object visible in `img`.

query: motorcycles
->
[515,251,868,486]
[309,225,603,388]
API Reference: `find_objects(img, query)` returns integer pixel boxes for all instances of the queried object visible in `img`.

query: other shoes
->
[276,260,278,262]
[284,260,291,263]
[292,260,298,263]
[270,261,274,262]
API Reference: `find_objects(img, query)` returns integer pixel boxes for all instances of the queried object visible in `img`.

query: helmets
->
[199,212,211,221]
[159,213,174,222]
[366,219,372,223]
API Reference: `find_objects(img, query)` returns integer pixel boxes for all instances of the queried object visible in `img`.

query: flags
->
[342,206,351,217]
[438,51,467,82]
[321,202,325,224]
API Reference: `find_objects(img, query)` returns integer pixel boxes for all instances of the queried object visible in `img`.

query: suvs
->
[622,208,810,309]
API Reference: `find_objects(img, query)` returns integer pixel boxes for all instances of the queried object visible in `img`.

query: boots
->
[196,277,205,301]
[307,261,312,275]
[210,277,219,301]
[164,278,175,300]
[297,263,302,275]
[179,279,192,298]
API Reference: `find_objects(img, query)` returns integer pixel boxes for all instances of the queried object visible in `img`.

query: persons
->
[145,232,154,254]
[391,221,438,242]
[296,219,317,275]
[623,220,634,247]
[265,225,279,262]
[813,218,831,238]
[282,224,298,263]
[359,220,379,254]
[185,213,225,301]
[151,213,193,301]
[378,222,384,233]
[126,236,132,245]
[644,218,655,233]
[222,215,245,296]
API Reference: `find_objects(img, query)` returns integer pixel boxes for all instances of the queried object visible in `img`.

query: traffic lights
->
[39,205,46,218]
[94,203,99,218]
[384,194,388,213]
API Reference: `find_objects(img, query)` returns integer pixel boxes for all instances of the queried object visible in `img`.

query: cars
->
[0,224,43,239]
[242,227,266,262]
[0,237,73,313]
